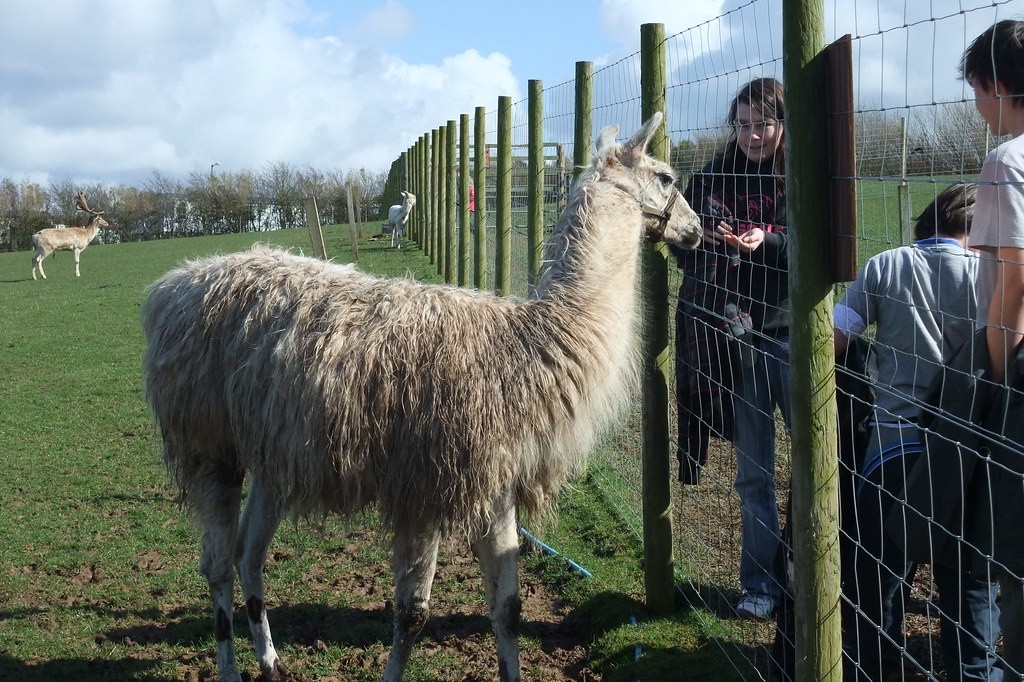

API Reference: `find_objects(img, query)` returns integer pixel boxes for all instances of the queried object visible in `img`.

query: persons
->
[469,175,474,234]
[668,77,790,625]
[835,181,981,682]
[955,21,1024,682]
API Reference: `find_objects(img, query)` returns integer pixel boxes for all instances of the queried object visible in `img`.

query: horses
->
[388,191,416,250]
[143,112,704,682]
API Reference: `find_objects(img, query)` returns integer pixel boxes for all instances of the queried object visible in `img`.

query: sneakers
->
[735,593,780,619]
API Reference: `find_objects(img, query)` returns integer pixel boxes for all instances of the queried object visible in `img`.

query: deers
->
[30,192,110,280]
